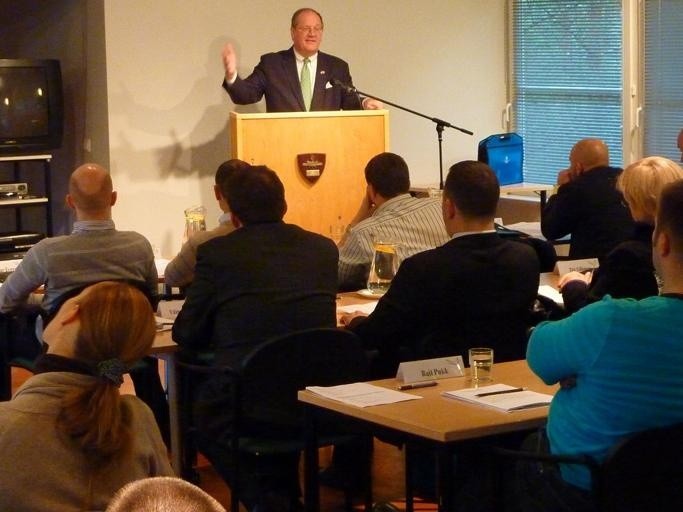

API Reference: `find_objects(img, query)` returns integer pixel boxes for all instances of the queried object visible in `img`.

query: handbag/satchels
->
[479,133,523,185]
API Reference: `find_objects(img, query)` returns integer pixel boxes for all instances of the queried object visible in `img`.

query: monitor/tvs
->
[0,57,66,156]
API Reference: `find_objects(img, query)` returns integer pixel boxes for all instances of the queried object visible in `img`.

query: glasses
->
[621,200,632,207]
[292,25,324,32]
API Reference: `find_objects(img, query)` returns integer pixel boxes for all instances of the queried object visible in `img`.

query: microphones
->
[329,78,359,96]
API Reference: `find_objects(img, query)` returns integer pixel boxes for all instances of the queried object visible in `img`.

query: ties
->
[300,58,312,112]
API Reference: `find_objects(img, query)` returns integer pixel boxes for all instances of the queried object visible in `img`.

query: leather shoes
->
[320,465,363,492]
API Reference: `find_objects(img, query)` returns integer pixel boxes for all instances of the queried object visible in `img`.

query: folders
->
[478,132,524,187]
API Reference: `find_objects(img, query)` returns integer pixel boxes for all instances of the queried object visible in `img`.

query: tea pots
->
[181,205,207,250]
[366,239,410,294]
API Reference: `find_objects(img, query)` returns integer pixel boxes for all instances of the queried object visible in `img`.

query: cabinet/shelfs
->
[0,154,51,284]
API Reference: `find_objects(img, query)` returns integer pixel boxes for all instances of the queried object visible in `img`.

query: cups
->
[470,348,493,383]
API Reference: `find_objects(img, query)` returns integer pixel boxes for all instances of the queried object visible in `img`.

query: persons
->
[0,281,175,512]
[338,153,451,290]
[558,156,683,313]
[222,8,384,113]
[165,159,252,287]
[319,160,540,492]
[514,178,683,512]
[171,165,340,512]
[543,139,653,260]
[0,163,158,359]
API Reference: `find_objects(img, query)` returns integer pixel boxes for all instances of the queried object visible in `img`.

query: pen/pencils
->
[475,387,529,397]
[335,296,344,299]
[398,381,439,390]
[163,323,174,325]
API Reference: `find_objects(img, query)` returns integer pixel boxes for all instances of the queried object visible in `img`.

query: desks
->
[297,360,559,512]
[138,288,390,488]
[495,182,555,224]
[233,110,388,247]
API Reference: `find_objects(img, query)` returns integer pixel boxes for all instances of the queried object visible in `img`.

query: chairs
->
[483,427,683,512]
[177,329,355,511]
